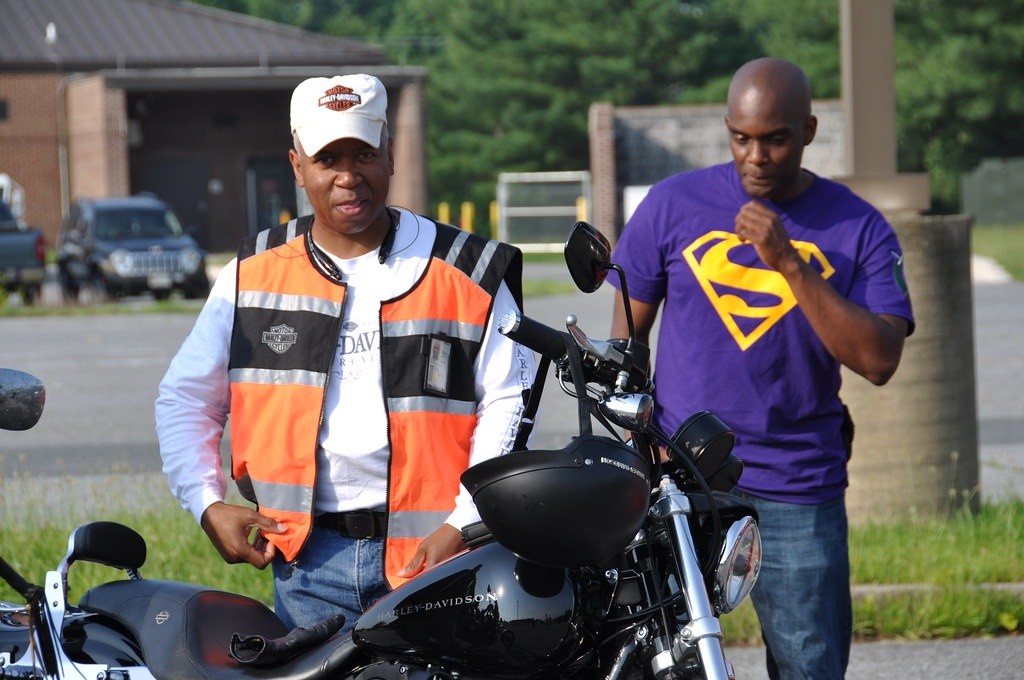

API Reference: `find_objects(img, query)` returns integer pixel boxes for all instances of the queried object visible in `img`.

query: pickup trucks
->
[0,197,45,305]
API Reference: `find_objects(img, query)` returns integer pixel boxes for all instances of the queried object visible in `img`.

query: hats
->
[289,74,388,157]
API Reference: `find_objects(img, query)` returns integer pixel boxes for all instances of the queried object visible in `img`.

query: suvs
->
[56,193,209,303]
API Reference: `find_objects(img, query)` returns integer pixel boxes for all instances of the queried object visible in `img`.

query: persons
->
[605,57,916,680]
[155,73,540,636]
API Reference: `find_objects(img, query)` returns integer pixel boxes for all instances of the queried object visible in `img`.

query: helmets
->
[459,435,651,573]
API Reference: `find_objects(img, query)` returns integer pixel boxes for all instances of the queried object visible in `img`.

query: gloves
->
[228,615,345,669]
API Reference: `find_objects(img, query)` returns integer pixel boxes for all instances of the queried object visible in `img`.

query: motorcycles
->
[42,220,764,680]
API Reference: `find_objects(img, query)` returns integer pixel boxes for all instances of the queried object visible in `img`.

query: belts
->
[312,508,390,539]
